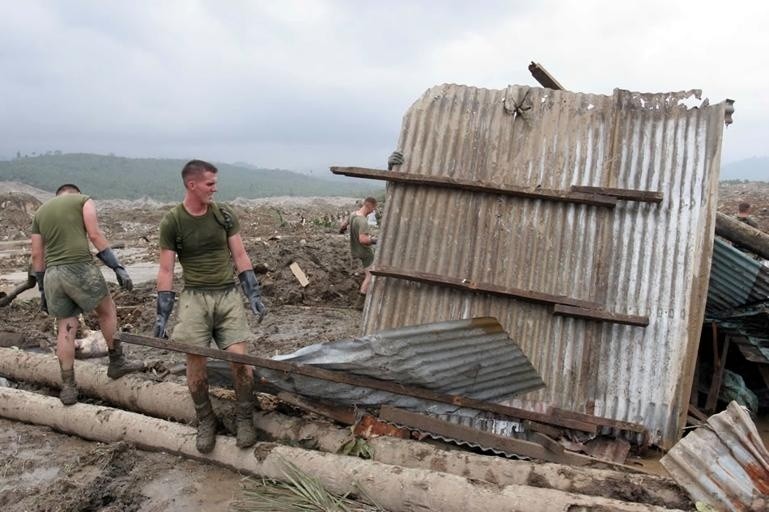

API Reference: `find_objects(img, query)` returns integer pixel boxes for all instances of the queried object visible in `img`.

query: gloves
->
[238,266,269,324]
[32,270,51,317]
[96,244,134,294]
[150,288,178,340]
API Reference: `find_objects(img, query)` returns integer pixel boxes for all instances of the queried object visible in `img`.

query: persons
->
[385,150,405,192]
[732,203,761,262]
[30,182,146,408]
[338,194,378,311]
[153,160,265,454]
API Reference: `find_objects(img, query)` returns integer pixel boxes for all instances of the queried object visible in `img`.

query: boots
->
[230,398,260,448]
[107,348,146,380]
[353,294,366,310]
[59,381,80,407]
[190,396,223,454]
